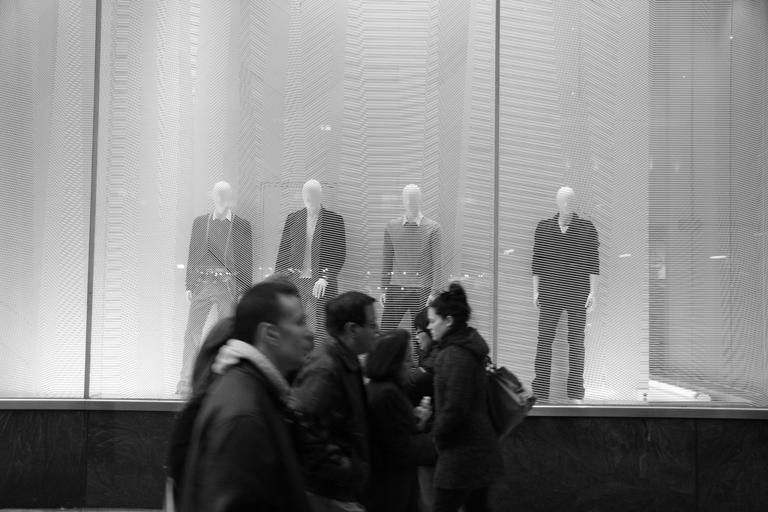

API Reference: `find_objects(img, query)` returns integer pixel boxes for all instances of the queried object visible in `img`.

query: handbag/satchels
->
[486,366,537,442]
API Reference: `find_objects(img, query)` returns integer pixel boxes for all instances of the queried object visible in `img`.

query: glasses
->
[416,331,427,334]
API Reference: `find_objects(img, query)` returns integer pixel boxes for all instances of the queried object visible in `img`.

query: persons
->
[275,179,347,349]
[381,184,444,357]
[531,187,600,406]
[175,181,253,400]
[163,284,508,512]
